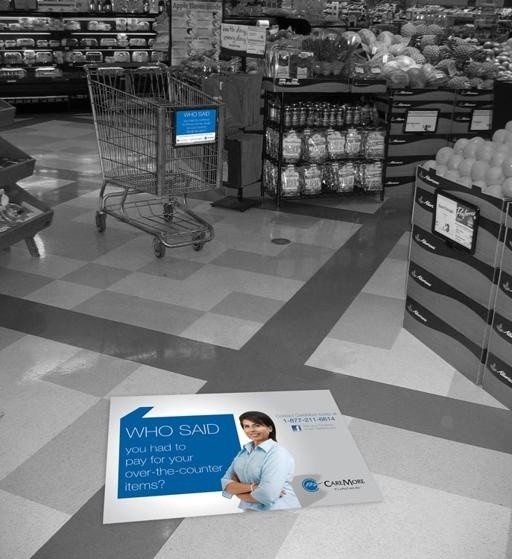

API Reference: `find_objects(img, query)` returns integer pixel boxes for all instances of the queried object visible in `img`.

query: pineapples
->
[401,23,496,91]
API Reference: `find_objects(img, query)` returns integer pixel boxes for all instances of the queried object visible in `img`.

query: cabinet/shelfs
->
[0,0,512,416]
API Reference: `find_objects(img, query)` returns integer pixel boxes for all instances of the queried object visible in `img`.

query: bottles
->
[89,0,112,12]
[143,0,165,14]
[270,101,378,127]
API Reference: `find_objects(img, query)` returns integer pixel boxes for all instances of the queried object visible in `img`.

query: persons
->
[220,411,302,511]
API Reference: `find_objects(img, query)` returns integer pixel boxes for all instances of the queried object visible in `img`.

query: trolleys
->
[80,54,228,260]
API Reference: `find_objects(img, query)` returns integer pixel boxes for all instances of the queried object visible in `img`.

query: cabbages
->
[341,29,434,90]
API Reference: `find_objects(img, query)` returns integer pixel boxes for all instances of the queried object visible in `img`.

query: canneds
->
[268,101,374,127]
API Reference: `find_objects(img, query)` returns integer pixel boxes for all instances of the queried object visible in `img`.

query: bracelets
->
[251,483,257,492]
